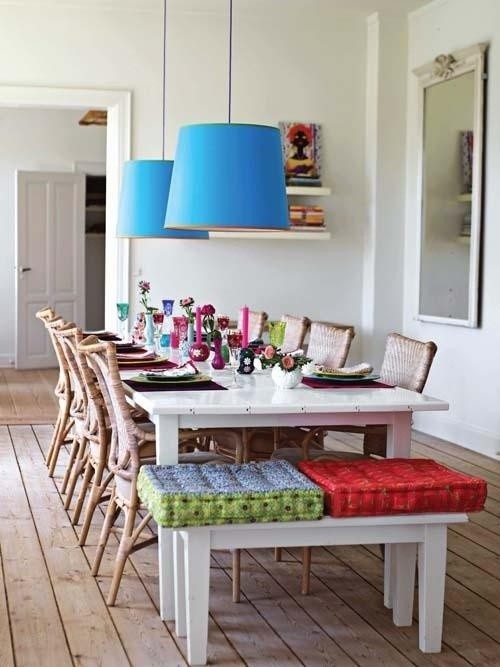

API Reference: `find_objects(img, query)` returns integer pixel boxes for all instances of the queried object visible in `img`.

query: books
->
[289,202,325,228]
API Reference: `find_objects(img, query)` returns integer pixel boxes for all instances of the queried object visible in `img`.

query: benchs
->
[158,513,468,667]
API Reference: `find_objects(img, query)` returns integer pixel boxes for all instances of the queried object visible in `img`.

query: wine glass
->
[227,328,242,348]
[226,348,244,390]
[116,303,128,343]
[154,300,175,337]
[217,315,229,342]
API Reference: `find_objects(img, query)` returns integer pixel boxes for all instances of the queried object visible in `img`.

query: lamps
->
[164,0,289,230]
[116,0,210,239]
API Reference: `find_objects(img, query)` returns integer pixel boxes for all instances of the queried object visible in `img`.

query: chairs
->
[222,309,427,596]
[36,310,243,605]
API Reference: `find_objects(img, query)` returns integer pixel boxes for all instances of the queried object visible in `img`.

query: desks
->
[80,330,449,620]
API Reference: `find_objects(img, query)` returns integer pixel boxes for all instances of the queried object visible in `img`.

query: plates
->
[117,357,167,364]
[304,369,381,382]
[128,374,213,384]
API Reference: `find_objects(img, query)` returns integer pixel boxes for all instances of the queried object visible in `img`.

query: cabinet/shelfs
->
[208,187,332,241]
[457,193,471,244]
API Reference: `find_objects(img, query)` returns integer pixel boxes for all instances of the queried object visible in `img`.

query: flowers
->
[138,280,150,313]
[181,297,194,323]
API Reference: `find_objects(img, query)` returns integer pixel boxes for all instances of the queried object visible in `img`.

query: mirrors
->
[413,42,489,329]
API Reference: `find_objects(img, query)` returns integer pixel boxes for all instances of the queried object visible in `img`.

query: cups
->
[269,321,286,346]
[160,329,180,348]
